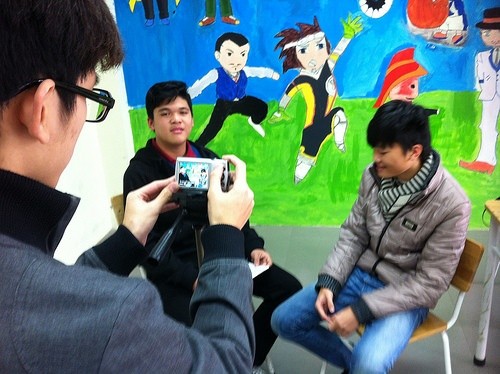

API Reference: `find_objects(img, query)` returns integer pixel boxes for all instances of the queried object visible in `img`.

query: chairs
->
[356,236,485,374]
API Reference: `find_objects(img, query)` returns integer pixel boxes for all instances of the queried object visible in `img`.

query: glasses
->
[8,78,114,122]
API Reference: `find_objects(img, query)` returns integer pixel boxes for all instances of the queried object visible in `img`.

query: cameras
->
[175,157,230,192]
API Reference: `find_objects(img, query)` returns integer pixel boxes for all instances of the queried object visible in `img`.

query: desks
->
[473,200,500,367]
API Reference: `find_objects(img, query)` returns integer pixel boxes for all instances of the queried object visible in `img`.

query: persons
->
[0,0,256,374]
[124,80,303,374]
[179,166,207,187]
[271,99,472,373]
